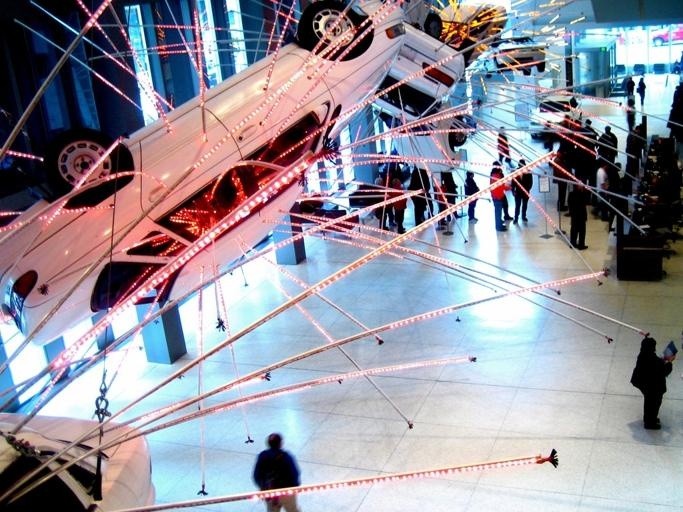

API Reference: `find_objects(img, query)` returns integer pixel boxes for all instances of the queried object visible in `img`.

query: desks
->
[635,134,683,240]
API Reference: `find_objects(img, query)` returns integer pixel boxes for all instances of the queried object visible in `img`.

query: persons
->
[544,115,636,250]
[628,335,679,429]
[251,433,301,511]
[624,76,647,178]
[371,152,480,234]
[491,127,534,231]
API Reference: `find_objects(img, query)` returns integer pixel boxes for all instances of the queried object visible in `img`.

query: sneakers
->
[500,215,529,231]
[383,221,406,235]
[644,417,661,430]
[572,243,588,250]
[429,213,460,224]
[470,217,479,222]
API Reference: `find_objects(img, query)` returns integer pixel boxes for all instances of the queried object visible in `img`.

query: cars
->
[651,24,683,47]
[0,412,157,512]
[0,1,547,347]
[528,94,583,137]
